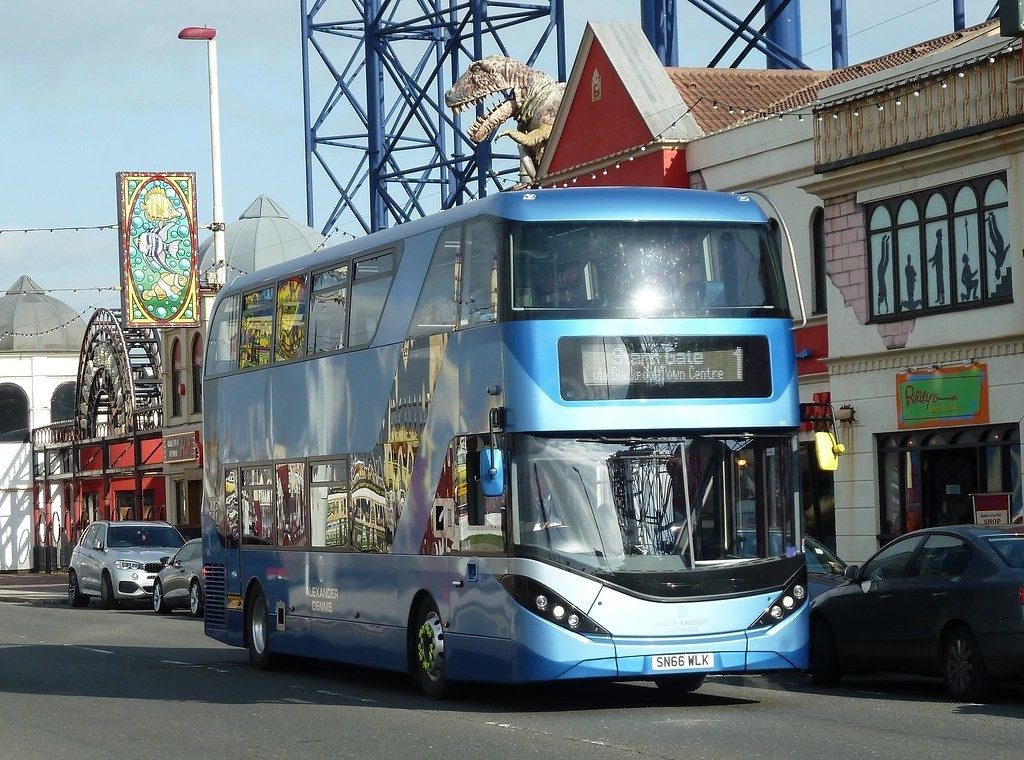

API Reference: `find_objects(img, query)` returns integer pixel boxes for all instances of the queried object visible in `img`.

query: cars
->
[153,538,203,618]
[736,529,852,601]
[809,525,1024,700]
[68,520,186,609]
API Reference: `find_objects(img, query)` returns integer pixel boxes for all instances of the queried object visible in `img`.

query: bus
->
[193,187,845,701]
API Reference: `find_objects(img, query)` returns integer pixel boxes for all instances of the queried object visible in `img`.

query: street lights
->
[179,21,226,295]
